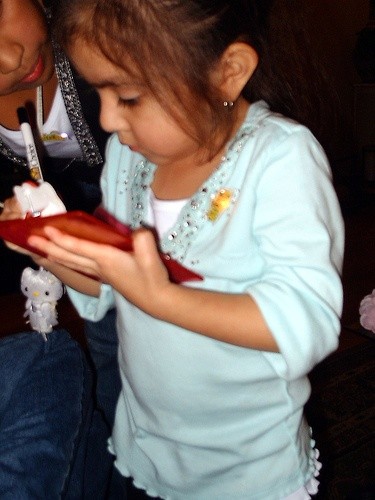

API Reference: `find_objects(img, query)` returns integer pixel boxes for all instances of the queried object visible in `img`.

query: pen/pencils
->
[17,105,44,186]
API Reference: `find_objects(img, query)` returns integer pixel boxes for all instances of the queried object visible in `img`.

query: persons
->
[0,1,109,275]
[30,1,344,499]
